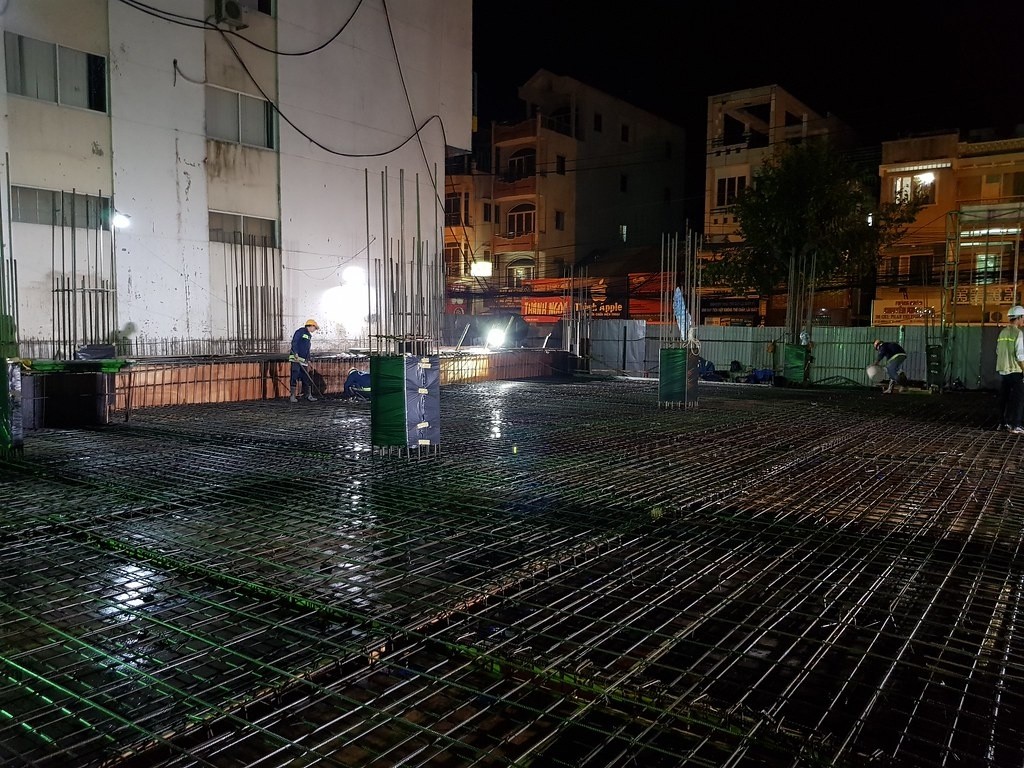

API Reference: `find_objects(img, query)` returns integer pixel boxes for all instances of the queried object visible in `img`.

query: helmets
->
[1007,305,1024,320]
[874,340,884,349]
[306,319,319,330]
[347,367,358,375]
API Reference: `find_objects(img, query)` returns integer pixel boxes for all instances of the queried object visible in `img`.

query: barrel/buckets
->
[866,363,884,382]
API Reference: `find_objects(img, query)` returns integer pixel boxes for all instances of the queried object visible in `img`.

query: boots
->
[882,379,894,393]
[898,386,908,392]
[305,386,317,401]
[289,385,298,403]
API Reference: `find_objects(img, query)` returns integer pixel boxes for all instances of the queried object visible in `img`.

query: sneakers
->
[997,424,1012,431]
[1010,426,1024,434]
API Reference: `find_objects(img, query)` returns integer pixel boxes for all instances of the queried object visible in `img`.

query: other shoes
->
[345,396,370,404]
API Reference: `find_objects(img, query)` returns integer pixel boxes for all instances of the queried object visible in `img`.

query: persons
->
[699,357,715,380]
[342,366,371,402]
[288,319,320,402]
[993,306,1024,434]
[868,339,908,394]
